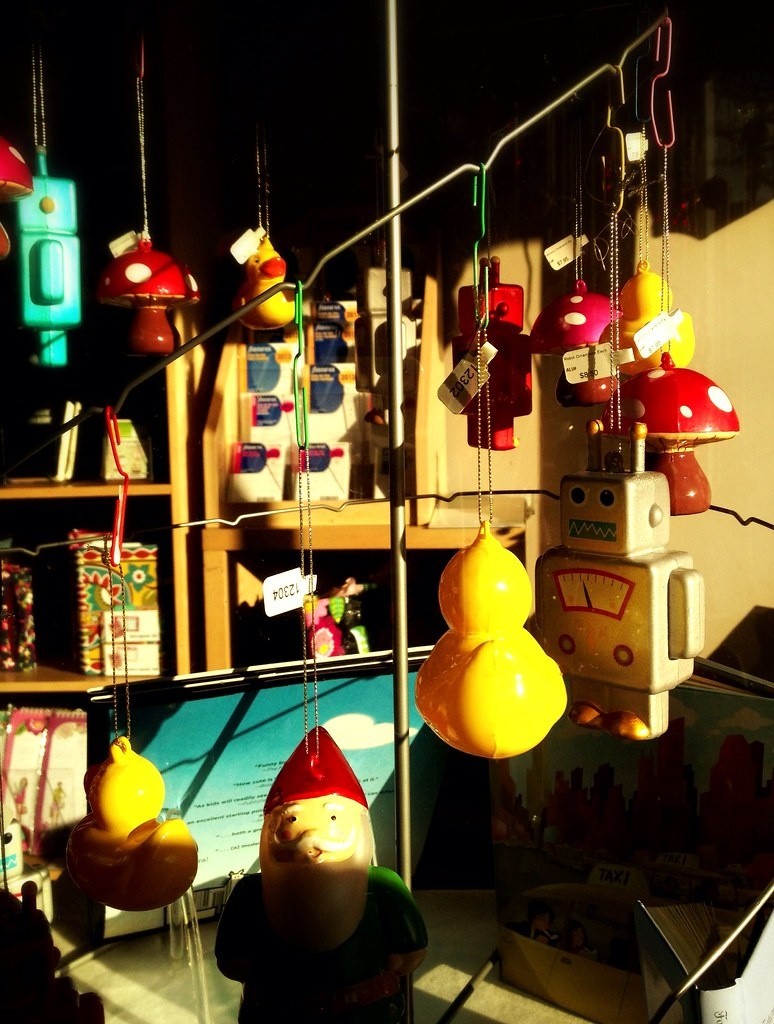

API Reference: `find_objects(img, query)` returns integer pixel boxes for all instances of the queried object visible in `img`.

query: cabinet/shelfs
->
[0,294,544,694]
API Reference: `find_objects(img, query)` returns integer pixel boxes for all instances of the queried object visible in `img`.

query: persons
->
[215,726,428,1024]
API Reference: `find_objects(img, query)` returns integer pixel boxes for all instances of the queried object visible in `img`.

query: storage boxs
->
[497,882,697,1024]
